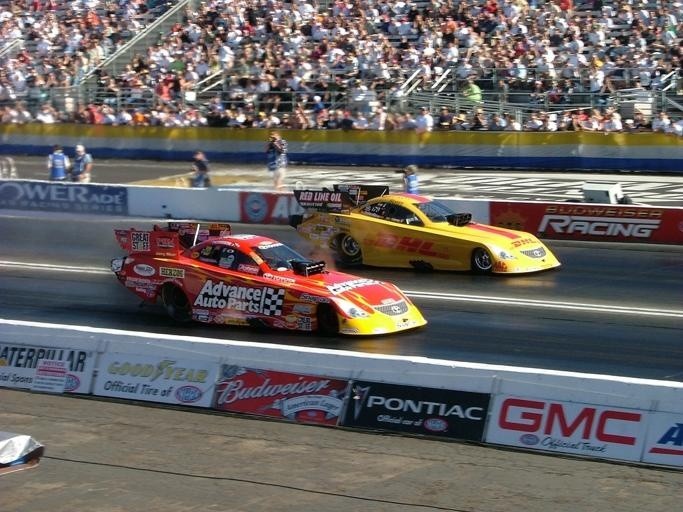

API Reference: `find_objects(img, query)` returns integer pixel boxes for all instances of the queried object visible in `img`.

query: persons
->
[190,150,211,187]
[0,0,683,146]
[46,143,71,182]
[401,164,419,195]
[265,131,287,190]
[67,144,93,183]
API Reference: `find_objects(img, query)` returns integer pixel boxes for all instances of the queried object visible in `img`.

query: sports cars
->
[107,212,433,340]
[280,180,566,281]
